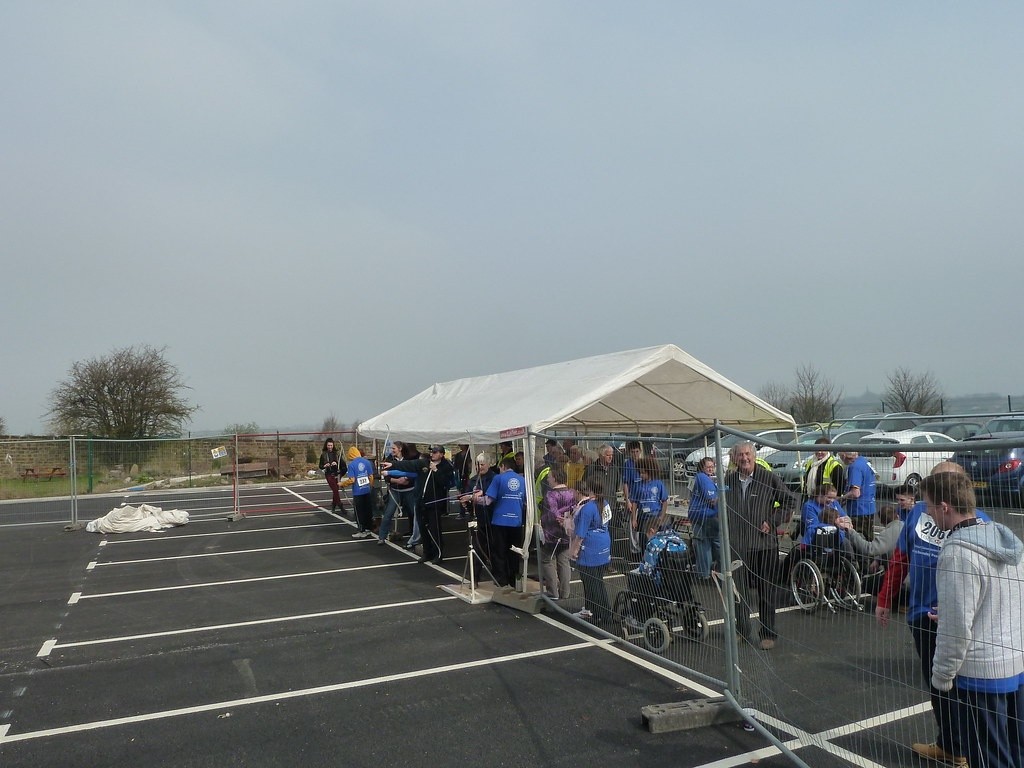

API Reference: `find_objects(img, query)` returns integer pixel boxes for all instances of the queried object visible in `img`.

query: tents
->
[355,343,804,597]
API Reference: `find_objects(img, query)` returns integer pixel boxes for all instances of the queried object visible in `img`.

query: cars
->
[685,428,806,477]
[857,431,956,490]
[764,428,874,486]
[911,422,982,442]
[951,431,1024,507]
[976,417,1024,434]
[650,431,723,478]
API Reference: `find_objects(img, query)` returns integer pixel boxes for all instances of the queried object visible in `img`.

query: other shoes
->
[432,558,442,564]
[352,530,372,539]
[609,566,618,575]
[573,609,592,619]
[377,539,385,544]
[862,569,883,579]
[418,555,430,563]
[757,640,775,650]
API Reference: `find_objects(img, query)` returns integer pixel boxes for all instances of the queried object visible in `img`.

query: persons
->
[319,438,348,515]
[337,441,477,565]
[625,517,689,629]
[460,440,669,625]
[688,438,917,650]
[875,461,1024,768]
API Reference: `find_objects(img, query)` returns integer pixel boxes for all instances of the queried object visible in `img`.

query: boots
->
[331,502,336,514]
[338,503,347,514]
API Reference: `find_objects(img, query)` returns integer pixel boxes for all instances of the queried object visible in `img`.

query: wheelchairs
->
[613,550,709,651]
[789,528,864,615]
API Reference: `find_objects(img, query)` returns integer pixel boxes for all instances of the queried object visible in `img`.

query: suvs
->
[838,412,926,434]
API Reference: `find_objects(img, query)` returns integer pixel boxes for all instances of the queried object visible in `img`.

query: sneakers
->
[912,742,967,766]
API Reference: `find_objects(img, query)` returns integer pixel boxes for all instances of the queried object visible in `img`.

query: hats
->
[428,446,445,452]
